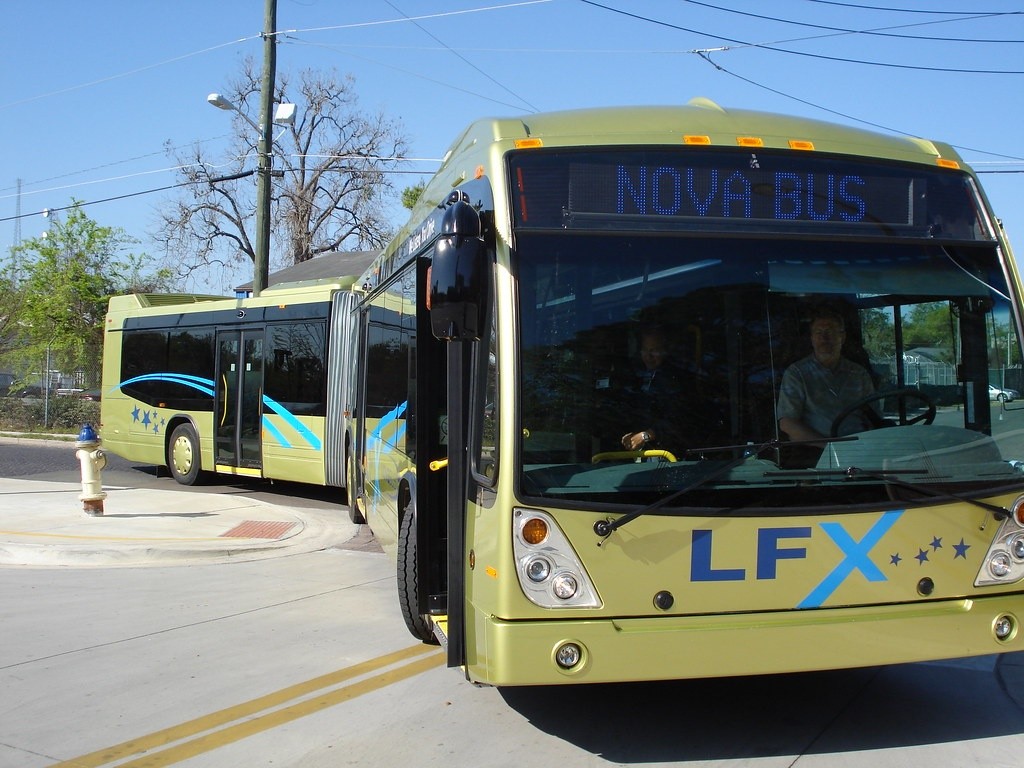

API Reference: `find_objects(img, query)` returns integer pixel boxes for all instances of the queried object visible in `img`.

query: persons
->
[599,329,696,452]
[771,308,886,448]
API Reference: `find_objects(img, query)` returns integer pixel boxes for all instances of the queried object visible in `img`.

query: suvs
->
[0,372,52,403]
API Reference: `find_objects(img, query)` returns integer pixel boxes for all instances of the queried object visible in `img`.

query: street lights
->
[206,93,266,141]
[272,103,297,145]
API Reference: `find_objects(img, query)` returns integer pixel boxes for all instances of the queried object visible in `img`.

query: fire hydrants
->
[75,424,107,518]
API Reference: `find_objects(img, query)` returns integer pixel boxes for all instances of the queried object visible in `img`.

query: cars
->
[989,384,1020,401]
[80,387,101,402]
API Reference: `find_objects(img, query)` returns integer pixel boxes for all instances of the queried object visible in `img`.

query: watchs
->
[642,430,651,443]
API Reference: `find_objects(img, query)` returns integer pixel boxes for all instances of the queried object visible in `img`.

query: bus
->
[94,98,1024,695]
